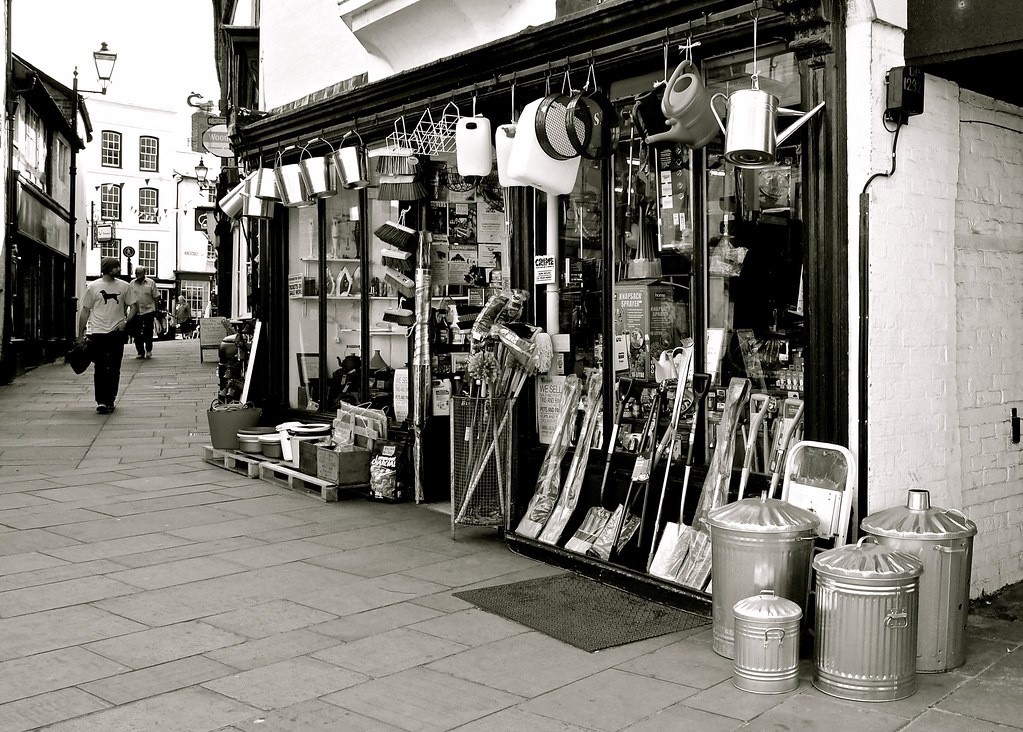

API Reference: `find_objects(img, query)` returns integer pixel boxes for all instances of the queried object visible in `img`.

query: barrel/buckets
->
[216,130,373,221]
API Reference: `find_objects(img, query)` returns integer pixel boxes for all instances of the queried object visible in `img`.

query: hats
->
[101,257,120,275]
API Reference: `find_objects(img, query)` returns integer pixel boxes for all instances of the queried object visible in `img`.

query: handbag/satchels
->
[65,337,91,375]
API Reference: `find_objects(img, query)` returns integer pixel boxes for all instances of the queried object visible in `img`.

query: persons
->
[129,266,160,359]
[77,258,139,414]
[126,295,197,344]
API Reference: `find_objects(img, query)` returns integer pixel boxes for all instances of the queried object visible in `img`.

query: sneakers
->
[145,351,152,358]
[135,354,143,359]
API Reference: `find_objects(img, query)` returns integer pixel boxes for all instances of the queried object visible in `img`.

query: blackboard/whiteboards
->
[200,317,229,349]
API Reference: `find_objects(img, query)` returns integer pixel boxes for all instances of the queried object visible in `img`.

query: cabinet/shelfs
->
[299,259,409,338]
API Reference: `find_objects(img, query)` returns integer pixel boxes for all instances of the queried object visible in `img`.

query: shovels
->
[514,375,582,540]
[564,377,636,554]
[590,344,805,596]
[536,374,605,547]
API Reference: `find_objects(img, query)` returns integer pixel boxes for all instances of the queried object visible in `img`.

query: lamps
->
[195,156,216,191]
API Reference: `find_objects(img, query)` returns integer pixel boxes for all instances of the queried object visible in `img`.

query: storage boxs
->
[298,439,372,485]
[614,278,674,382]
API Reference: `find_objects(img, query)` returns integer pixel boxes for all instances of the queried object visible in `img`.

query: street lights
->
[64,43,119,365]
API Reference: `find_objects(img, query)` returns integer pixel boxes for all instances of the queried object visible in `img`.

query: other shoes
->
[187,337,189,339]
[95,404,114,413]
[183,337,186,340]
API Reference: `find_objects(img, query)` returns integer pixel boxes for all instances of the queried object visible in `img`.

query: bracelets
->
[124,320,127,323]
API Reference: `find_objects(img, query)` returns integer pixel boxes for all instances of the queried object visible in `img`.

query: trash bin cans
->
[700,488,978,703]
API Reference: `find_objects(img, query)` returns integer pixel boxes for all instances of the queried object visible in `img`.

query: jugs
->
[336,344,362,371]
[709,75,826,169]
[644,60,723,150]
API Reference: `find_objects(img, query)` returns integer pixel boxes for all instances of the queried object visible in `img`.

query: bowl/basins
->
[236,425,281,458]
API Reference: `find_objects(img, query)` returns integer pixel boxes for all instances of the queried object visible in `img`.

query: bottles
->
[495,97,585,196]
[456,116,492,177]
[370,350,387,369]
